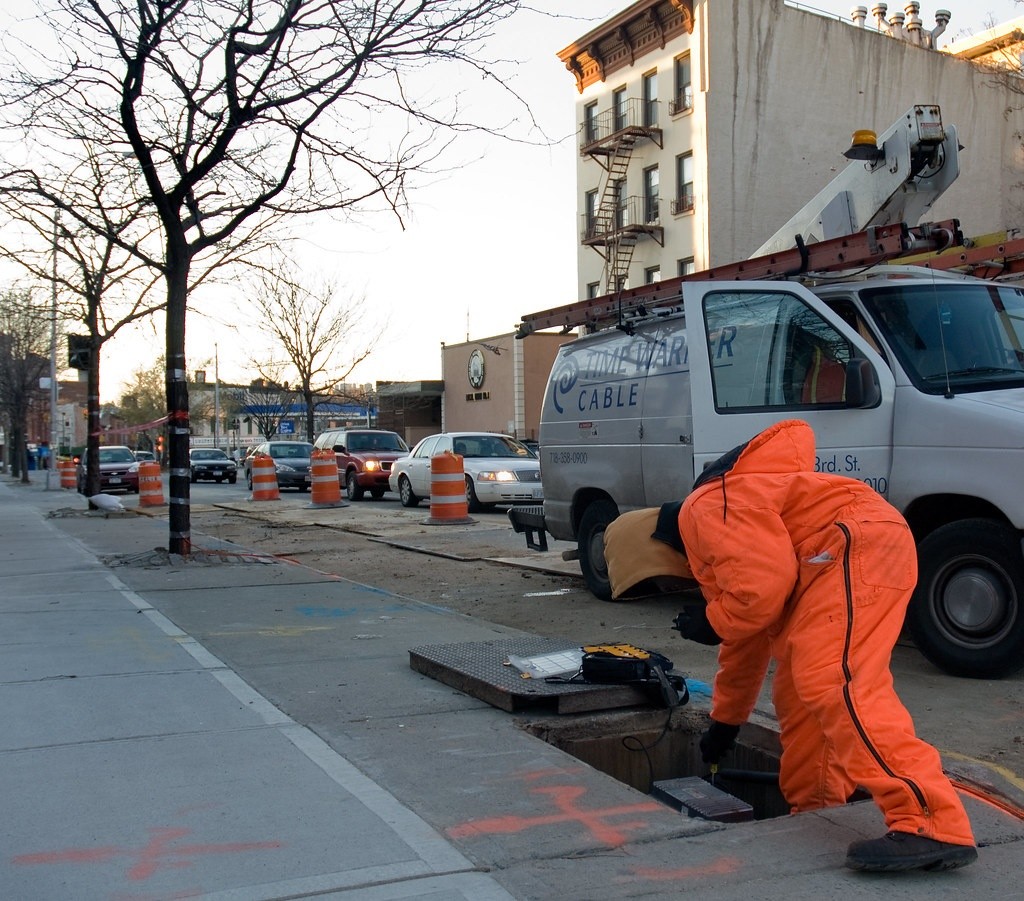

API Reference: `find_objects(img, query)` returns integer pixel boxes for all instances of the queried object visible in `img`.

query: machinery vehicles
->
[507,106,1023,678]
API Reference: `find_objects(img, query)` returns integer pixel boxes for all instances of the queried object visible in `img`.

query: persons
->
[606,419,979,876]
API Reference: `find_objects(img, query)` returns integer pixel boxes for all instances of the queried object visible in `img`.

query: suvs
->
[306,426,410,501]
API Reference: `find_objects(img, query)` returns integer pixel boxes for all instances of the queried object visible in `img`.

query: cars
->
[189,448,238,484]
[126,451,156,462]
[229,449,246,466]
[76,446,140,496]
[245,444,260,456]
[244,441,314,492]
[388,432,544,513]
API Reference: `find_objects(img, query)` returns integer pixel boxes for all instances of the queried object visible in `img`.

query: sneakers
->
[844,831,978,874]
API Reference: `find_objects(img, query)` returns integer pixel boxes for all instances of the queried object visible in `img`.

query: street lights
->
[62,412,65,450]
[46,151,137,489]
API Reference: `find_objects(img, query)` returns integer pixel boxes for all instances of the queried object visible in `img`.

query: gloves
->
[699,720,740,764]
[681,605,723,646]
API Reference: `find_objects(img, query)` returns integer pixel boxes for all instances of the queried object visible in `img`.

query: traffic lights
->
[157,436,164,450]
